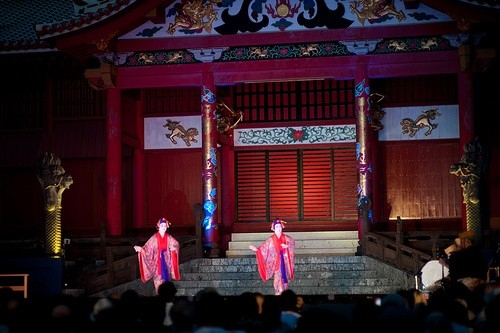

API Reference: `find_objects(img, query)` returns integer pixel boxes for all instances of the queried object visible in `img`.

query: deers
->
[350,3,404,28]
[166,11,218,35]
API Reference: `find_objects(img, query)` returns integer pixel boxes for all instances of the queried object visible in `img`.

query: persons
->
[0,254,500,332]
[135,218,181,292]
[250,219,295,294]
